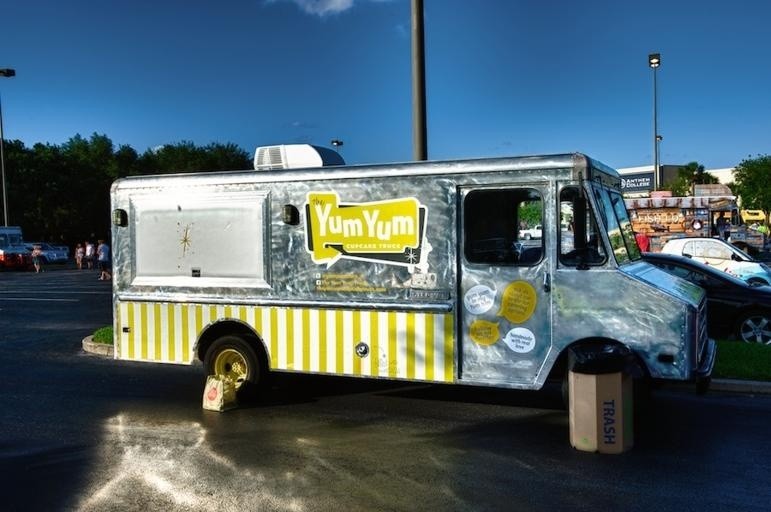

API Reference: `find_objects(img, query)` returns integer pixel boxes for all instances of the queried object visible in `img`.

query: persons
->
[96,240,111,281]
[31,245,42,274]
[74,243,85,269]
[83,239,94,268]
[717,210,732,241]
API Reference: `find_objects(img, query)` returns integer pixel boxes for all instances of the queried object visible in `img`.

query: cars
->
[641,253,771,346]
[26,242,68,264]
[660,236,771,291]
[518,223,544,241]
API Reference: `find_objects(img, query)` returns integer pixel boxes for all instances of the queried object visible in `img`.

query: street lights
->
[0,68,16,227]
[648,53,660,191]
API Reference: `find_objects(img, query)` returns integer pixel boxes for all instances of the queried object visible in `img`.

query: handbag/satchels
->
[202,375,237,412]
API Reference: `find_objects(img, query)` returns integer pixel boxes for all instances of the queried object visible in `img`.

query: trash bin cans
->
[568,342,634,454]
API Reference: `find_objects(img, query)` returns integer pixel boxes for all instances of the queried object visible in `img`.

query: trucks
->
[0,227,33,272]
[110,145,719,411]
[623,195,766,257]
